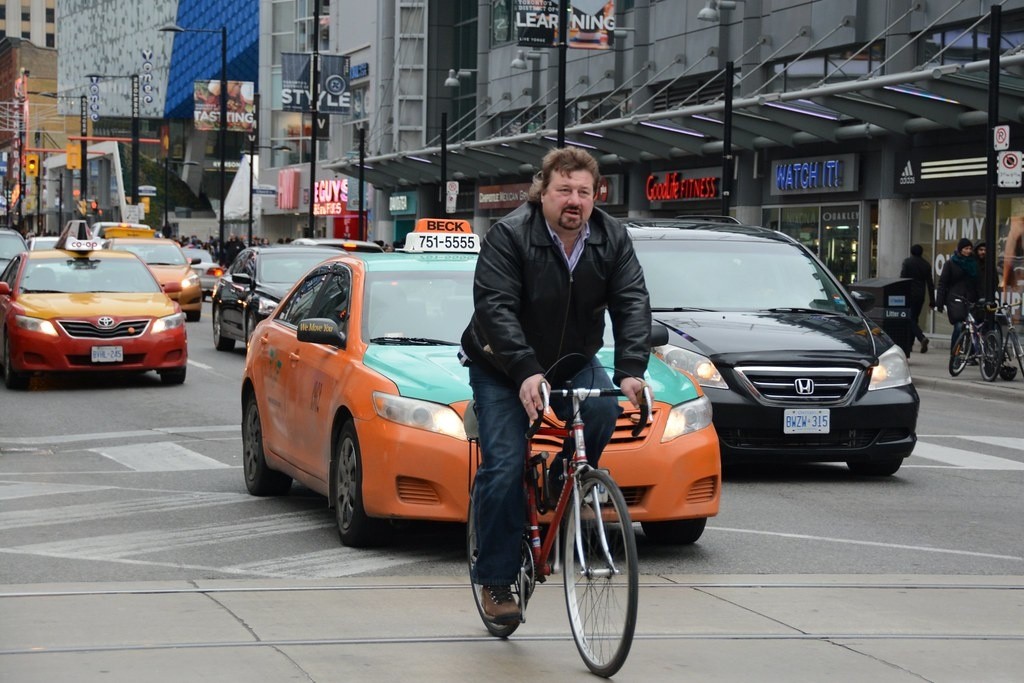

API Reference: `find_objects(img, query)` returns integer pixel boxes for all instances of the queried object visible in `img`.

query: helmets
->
[1000,365,1016,379]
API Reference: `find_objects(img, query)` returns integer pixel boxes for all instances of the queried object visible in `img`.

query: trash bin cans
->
[847,276,914,358]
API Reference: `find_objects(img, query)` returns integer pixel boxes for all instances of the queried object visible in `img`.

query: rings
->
[524,400,529,405]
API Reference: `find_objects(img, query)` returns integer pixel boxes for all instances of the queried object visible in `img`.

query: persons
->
[458,145,652,622]
[277,238,291,244]
[162,221,171,239]
[11,223,58,239]
[173,234,246,269]
[375,238,405,248]
[250,235,270,245]
[900,244,935,352]
[938,237,999,369]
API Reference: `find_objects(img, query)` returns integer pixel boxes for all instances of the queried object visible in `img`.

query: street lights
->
[83,71,142,207]
[246,141,292,244]
[163,159,200,226]
[157,23,228,247]
[34,90,88,221]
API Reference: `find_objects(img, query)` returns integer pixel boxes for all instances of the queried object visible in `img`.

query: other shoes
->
[966,359,978,366]
[920,338,929,352]
[953,356,961,369]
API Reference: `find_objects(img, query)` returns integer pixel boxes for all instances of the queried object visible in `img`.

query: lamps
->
[698,0,736,22]
[445,68,477,87]
[511,50,547,69]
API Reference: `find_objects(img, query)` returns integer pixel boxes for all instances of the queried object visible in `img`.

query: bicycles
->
[948,286,1024,384]
[462,353,655,679]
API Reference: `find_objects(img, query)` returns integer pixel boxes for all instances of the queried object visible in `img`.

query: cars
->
[24,235,62,252]
[240,219,722,550]
[289,236,388,280]
[0,220,189,389]
[181,247,226,299]
[211,243,352,355]
[0,229,29,284]
[90,222,153,238]
[101,226,203,320]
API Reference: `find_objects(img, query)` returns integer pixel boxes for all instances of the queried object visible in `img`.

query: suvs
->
[610,213,921,479]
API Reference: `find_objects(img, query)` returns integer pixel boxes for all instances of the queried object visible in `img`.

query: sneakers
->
[482,582,522,624]
[580,500,597,528]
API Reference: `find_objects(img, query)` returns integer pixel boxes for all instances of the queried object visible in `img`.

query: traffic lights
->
[26,154,38,177]
[86,197,99,215]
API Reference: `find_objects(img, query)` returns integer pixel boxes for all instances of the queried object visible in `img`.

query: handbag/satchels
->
[948,300,967,325]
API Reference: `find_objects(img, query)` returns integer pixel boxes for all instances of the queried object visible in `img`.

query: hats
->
[973,239,987,251]
[958,238,972,253]
